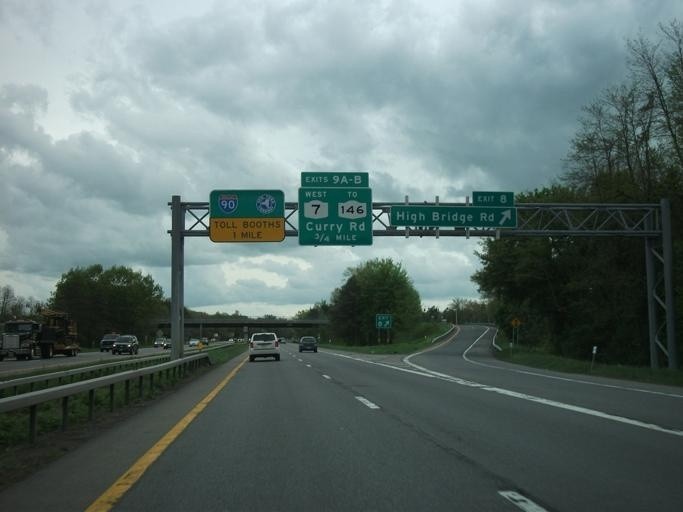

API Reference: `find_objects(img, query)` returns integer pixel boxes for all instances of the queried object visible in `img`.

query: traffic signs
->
[298,188,373,246]
[218,194,238,213]
[210,190,285,242]
[390,205,517,227]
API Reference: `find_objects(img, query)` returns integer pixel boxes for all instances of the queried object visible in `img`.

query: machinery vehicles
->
[1,318,43,360]
[37,309,79,359]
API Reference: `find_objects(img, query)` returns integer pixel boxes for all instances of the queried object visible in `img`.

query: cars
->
[248,332,281,361]
[189,337,208,348]
[154,337,172,348]
[298,337,317,353]
[99,333,119,352]
[112,336,139,355]
